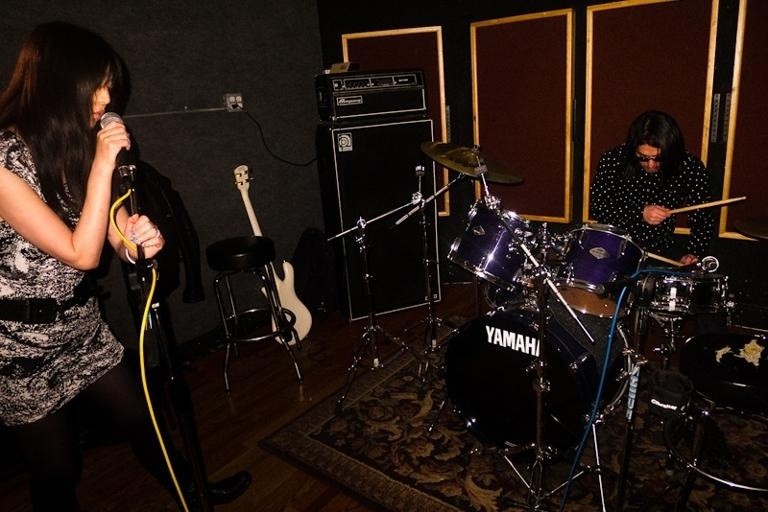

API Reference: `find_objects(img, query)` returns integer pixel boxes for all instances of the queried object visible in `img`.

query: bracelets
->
[124,245,138,267]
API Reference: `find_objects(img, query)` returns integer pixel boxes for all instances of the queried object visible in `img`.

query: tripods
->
[332,248,454,416]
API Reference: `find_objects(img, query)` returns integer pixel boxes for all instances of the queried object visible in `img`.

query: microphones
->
[101,111,135,179]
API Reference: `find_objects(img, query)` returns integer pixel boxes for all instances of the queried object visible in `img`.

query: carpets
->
[256,312,767,510]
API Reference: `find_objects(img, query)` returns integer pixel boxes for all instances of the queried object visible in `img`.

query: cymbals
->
[731,218,768,240]
[420,141,525,183]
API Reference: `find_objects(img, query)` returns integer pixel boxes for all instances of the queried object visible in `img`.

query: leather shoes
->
[174,470,252,512]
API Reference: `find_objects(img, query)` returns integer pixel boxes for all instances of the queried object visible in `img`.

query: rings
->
[687,255,692,261]
[152,225,162,237]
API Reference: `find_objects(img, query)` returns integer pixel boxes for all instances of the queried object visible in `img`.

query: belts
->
[0,277,91,325]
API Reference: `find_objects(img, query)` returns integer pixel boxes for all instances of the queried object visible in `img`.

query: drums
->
[444,291,629,463]
[651,274,732,316]
[448,203,532,293]
[552,222,644,320]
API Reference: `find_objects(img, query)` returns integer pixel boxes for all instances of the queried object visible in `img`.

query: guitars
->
[233,166,313,347]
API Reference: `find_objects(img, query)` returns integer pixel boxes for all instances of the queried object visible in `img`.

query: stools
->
[203,234,307,396]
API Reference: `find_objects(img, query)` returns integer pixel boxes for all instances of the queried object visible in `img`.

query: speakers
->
[314,117,443,325]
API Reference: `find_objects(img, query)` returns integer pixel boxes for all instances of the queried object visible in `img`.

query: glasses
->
[635,154,660,163]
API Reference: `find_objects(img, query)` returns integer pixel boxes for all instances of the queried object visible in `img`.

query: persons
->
[589,111,717,403]
[1,20,169,512]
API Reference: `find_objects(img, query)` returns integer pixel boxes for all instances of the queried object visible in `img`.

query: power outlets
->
[223,92,244,114]
